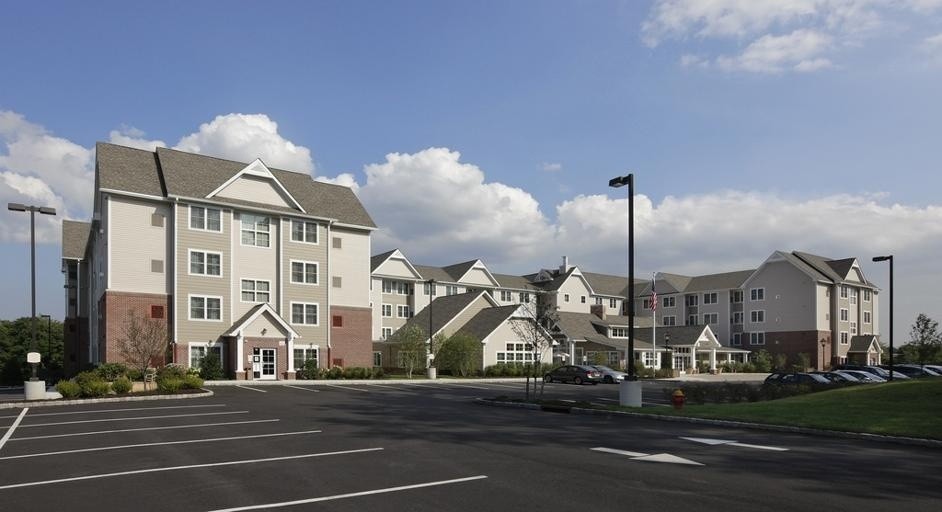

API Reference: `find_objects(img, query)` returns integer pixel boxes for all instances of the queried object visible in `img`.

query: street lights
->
[820,338,826,371]
[424,279,436,366]
[609,174,637,381]
[8,203,56,382]
[664,330,672,378]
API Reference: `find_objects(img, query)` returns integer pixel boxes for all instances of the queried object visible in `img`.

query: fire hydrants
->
[672,390,685,409]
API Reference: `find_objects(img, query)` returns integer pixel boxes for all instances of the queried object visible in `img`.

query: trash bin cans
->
[244,367,253,380]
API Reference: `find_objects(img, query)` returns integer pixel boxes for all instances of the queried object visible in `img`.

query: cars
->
[545,364,629,385]
[765,364,942,386]
[872,255,893,380]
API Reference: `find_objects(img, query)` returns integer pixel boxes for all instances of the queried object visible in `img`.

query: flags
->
[649,278,657,311]
